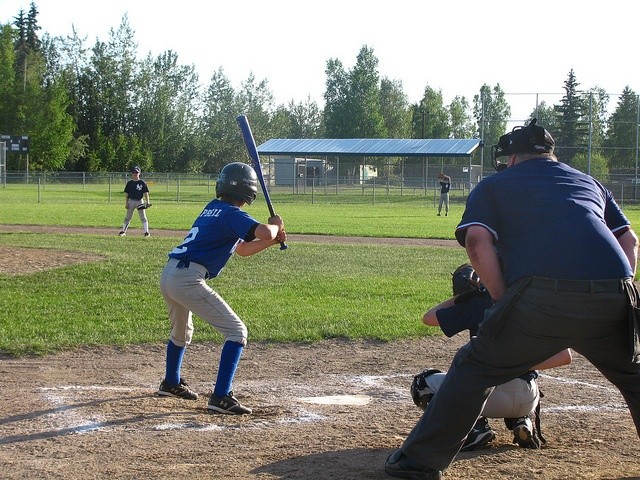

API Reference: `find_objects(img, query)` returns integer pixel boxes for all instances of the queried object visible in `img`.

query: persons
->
[157,161,288,416]
[385,124,640,480]
[409,261,573,453]
[437,174,451,216]
[118,166,152,237]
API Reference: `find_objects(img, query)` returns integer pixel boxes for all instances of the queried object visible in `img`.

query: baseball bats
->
[235,114,288,249]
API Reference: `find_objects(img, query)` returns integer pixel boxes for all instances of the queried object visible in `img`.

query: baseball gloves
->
[453,262,483,295]
[438,174,444,179]
[138,204,152,209]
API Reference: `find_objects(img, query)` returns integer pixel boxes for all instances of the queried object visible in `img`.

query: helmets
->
[491,118,556,172]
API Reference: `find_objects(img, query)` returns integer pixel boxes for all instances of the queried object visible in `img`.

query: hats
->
[132,166,141,172]
[215,163,258,202]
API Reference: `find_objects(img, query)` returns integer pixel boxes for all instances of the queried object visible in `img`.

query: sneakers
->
[513,417,541,449]
[207,391,252,415]
[462,416,495,451]
[158,379,198,400]
[119,231,126,236]
[385,450,443,480]
[144,232,150,237]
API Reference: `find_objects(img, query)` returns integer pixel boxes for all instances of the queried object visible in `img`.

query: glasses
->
[131,171,138,174]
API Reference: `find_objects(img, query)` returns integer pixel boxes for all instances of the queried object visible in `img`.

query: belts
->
[529,276,627,293]
[176,260,209,280]
